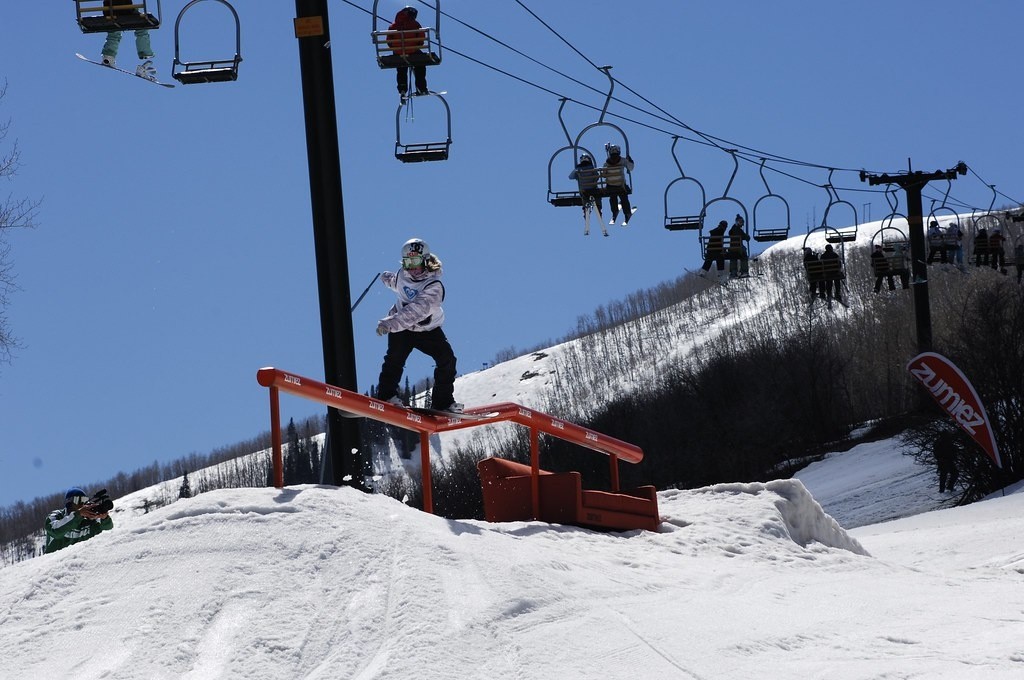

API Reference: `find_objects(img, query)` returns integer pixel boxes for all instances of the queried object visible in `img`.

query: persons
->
[871,245,909,292]
[44,487,114,554]
[1016,245,1024,277]
[973,229,1006,262]
[568,145,634,217]
[926,221,964,266]
[802,244,841,298]
[101,0,158,81]
[386,7,430,105]
[700,214,751,277]
[376,238,465,411]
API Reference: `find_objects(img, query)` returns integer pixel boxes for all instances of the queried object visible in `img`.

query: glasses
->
[402,254,430,268]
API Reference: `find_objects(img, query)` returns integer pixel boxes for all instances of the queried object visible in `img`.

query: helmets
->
[609,145,620,156]
[401,238,429,268]
[581,154,590,162]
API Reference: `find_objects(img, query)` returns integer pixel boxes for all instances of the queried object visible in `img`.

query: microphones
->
[91,489,107,499]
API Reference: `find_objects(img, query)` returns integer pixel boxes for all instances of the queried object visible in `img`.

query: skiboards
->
[608,200,638,226]
[74,53,176,89]
[806,288,850,309]
[584,195,610,238]
[918,259,969,277]
[871,280,927,295]
[398,90,448,107]
[718,273,765,279]
[683,267,730,289]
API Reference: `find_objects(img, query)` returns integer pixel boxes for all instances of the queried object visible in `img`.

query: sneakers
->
[103,55,114,65]
[139,52,155,59]
[447,403,464,410]
[387,395,402,404]
[400,91,406,104]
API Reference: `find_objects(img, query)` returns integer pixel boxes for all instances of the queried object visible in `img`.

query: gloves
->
[380,271,385,280]
[376,323,388,336]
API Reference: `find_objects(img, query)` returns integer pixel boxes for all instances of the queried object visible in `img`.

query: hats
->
[65,488,88,503]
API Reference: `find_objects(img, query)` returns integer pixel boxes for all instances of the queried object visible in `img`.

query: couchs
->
[478,457,660,532]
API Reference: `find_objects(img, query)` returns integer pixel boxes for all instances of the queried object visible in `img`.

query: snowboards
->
[339,407,500,421]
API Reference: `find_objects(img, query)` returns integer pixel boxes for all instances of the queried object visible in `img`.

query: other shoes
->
[700,269,706,276]
